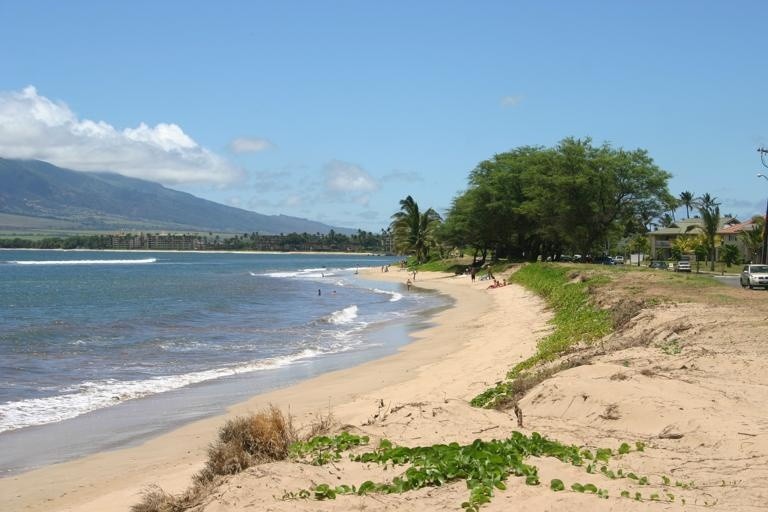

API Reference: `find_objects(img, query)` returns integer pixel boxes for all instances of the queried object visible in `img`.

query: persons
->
[381,264,389,273]
[318,289,321,296]
[400,260,404,270]
[470,267,476,282]
[488,265,495,280]
[413,269,417,280]
[406,278,410,292]
[487,279,511,290]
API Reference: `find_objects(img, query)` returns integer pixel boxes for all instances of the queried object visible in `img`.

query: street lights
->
[757,173,768,181]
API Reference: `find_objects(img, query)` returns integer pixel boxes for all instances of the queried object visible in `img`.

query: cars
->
[546,253,625,265]
[647,260,691,272]
[740,264,768,289]
